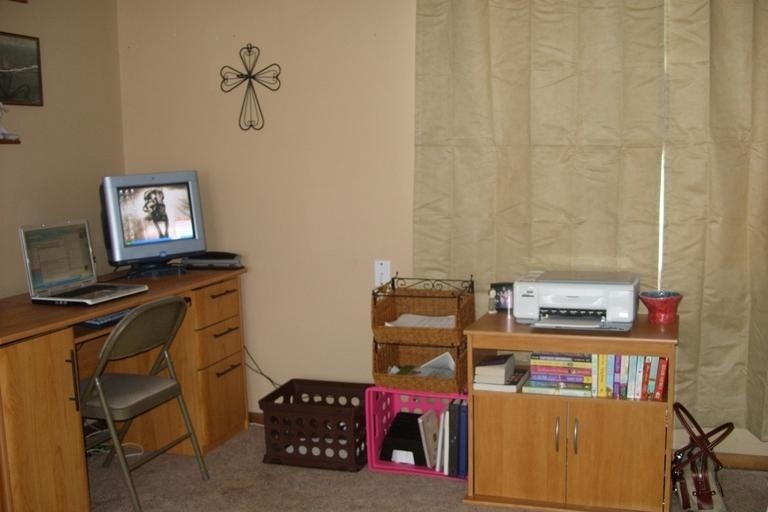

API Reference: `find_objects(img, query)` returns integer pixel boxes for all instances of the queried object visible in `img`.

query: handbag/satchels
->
[672,401,735,512]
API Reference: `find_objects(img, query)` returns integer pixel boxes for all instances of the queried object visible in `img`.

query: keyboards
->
[81,308,132,329]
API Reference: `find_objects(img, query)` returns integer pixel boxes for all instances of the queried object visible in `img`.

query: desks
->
[0,263,249,512]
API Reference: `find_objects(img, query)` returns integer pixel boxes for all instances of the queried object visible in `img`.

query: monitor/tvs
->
[99,170,207,280]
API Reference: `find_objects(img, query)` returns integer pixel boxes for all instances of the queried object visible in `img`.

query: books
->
[472,353,667,399]
[418,399,468,478]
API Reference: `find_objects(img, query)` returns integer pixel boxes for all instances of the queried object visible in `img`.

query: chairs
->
[79,294,209,512]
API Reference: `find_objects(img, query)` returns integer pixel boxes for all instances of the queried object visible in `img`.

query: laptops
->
[18,218,149,307]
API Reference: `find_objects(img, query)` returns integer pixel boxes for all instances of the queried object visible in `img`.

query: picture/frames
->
[0,31,44,107]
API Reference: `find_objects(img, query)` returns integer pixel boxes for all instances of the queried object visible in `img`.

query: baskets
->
[259,378,375,472]
[364,383,468,481]
[371,287,475,393]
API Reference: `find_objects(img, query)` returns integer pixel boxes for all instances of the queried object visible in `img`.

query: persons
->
[488,286,512,313]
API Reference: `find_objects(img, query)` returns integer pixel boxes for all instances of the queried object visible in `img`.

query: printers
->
[513,270,640,332]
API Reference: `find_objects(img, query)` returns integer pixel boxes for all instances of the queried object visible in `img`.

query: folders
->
[449,398,468,479]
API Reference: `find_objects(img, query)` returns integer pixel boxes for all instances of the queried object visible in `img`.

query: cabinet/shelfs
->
[372,272,475,392]
[462,312,680,512]
